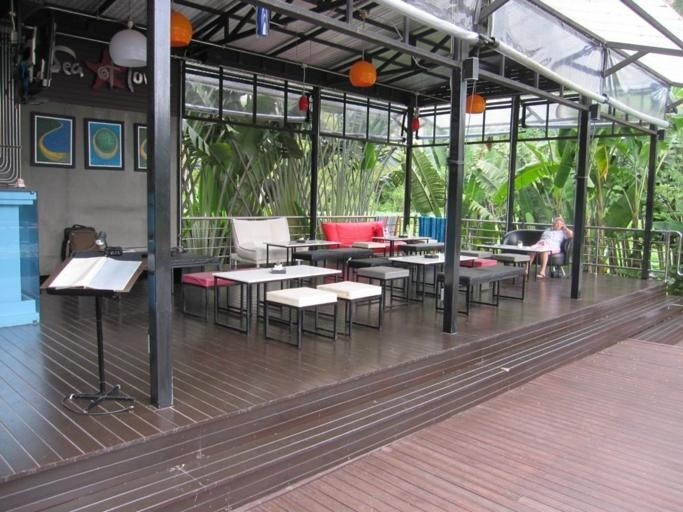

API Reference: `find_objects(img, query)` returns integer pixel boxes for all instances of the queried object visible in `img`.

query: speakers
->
[658,129,666,141]
[462,56,480,81]
[590,103,602,121]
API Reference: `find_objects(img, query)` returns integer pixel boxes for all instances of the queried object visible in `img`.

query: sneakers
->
[535,271,546,279]
[524,270,530,282]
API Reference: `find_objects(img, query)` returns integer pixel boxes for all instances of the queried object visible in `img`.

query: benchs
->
[435,265,526,315]
[230,216,309,269]
[292,248,373,283]
[460,256,496,298]
[320,222,407,256]
[499,229,573,278]
[397,242,445,255]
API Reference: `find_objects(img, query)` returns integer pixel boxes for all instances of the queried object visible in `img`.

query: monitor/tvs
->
[22,20,57,95]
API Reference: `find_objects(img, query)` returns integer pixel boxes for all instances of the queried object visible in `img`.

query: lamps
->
[466,47,486,113]
[170,1,193,47]
[411,91,421,132]
[299,65,309,111]
[349,11,376,87]
[95,231,122,256]
[109,0,148,67]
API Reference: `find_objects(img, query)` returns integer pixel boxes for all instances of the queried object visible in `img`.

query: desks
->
[105,250,220,308]
[212,264,343,336]
[387,254,478,301]
[261,240,341,270]
[372,236,429,256]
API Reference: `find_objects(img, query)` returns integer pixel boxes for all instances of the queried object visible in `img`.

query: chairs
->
[61,224,98,261]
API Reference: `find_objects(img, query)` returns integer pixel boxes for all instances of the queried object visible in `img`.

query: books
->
[48,257,143,291]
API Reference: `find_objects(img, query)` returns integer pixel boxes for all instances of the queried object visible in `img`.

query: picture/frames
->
[134,123,148,172]
[84,118,124,169]
[30,111,76,169]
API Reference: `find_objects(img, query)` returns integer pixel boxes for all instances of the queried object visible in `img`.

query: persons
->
[525,218,573,278]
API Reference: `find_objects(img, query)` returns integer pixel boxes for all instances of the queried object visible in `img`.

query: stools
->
[264,286,338,348]
[494,254,530,285]
[355,265,409,311]
[460,251,493,260]
[346,257,393,283]
[317,280,383,339]
[180,268,245,322]
[351,241,385,259]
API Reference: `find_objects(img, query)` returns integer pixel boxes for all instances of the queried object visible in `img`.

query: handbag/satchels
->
[63,223,100,258]
